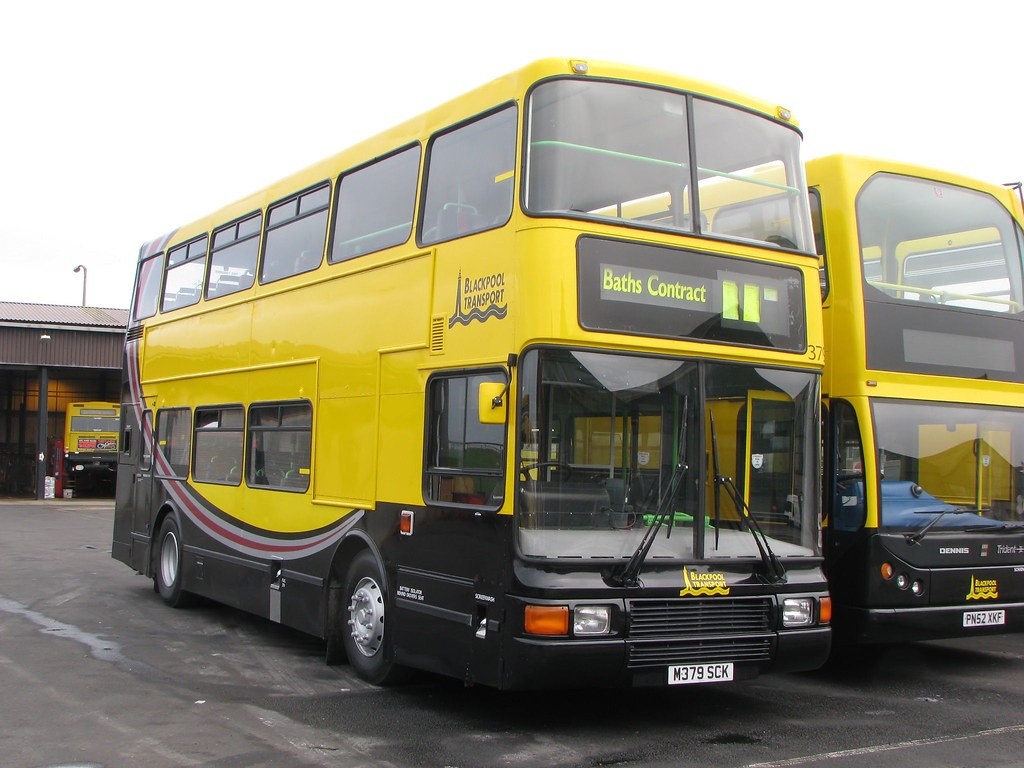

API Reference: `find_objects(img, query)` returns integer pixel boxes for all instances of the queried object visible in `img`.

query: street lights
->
[73,264,88,307]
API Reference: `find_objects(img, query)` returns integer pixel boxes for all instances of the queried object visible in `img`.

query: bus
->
[516,222,1024,546]
[521,154,1024,669]
[111,63,832,702]
[65,401,121,500]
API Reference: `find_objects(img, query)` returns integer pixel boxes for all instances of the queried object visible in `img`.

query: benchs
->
[161,199,484,315]
[198,447,311,492]
